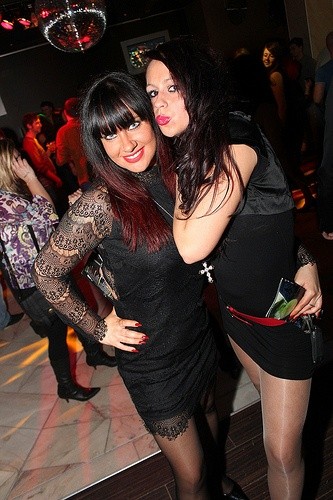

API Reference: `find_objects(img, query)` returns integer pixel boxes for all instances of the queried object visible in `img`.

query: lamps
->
[0,3,31,31]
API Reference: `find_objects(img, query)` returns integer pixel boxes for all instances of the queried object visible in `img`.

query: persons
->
[30,70,333,500]
[229,31,333,242]
[0,285,23,329]
[55,97,92,191]
[0,101,63,152]
[144,37,319,500]
[0,143,116,403]
[21,113,66,220]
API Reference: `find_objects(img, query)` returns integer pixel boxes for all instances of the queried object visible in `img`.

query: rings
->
[308,303,315,307]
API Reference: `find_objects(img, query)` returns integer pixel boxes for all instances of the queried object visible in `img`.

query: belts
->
[219,296,289,328]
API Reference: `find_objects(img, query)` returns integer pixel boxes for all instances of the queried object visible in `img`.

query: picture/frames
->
[119,29,171,76]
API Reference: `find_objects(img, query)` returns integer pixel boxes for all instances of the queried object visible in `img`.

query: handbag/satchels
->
[9,285,67,337]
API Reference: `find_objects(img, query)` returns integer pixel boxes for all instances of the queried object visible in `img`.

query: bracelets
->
[26,176,37,186]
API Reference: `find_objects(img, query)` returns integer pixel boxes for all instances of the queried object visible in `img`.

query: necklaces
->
[150,197,215,284]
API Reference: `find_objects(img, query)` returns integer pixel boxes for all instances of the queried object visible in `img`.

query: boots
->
[49,350,101,403]
[72,325,118,371]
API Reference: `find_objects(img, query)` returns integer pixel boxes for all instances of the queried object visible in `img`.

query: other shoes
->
[222,480,251,500]
[4,312,26,330]
